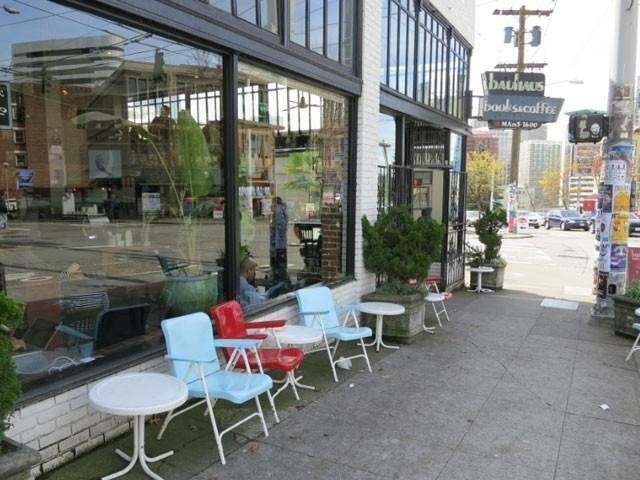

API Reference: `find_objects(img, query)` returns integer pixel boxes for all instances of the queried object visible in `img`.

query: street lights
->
[0,6,19,14]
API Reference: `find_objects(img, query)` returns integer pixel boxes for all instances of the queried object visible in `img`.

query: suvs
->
[6,197,18,211]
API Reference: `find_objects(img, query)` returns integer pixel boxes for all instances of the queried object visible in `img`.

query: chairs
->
[209,300,304,423]
[423,278,450,327]
[294,286,372,382]
[157,311,280,465]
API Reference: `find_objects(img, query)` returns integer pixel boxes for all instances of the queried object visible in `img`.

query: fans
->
[283,97,320,111]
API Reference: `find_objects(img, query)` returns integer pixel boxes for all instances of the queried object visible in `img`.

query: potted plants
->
[69,109,225,318]
[466,201,509,290]
[1,283,41,478]
[612,279,640,339]
[361,209,448,344]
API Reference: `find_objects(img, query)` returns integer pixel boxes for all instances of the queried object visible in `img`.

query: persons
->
[53,256,109,339]
[239,258,263,304]
[270,197,288,284]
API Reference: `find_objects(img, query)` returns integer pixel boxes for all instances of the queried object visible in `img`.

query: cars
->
[544,210,590,231]
[599,212,640,236]
[514,210,542,229]
[466,211,486,227]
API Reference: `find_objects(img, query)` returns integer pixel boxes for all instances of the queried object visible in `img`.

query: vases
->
[0,435,41,480]
[465,263,506,291]
[159,272,218,316]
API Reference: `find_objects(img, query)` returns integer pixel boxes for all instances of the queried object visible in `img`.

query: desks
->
[356,302,405,352]
[88,372,188,480]
[467,268,495,293]
[260,324,323,400]
